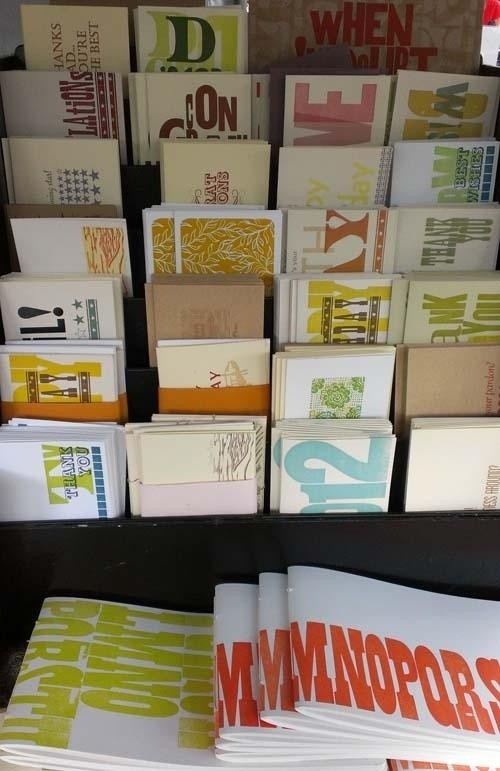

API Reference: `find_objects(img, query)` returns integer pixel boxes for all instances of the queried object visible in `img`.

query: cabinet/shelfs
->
[0,155,499,710]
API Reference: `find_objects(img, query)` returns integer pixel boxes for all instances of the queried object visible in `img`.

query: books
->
[2,566,500,771]
[1,411,499,521]
[1,1,500,423]
